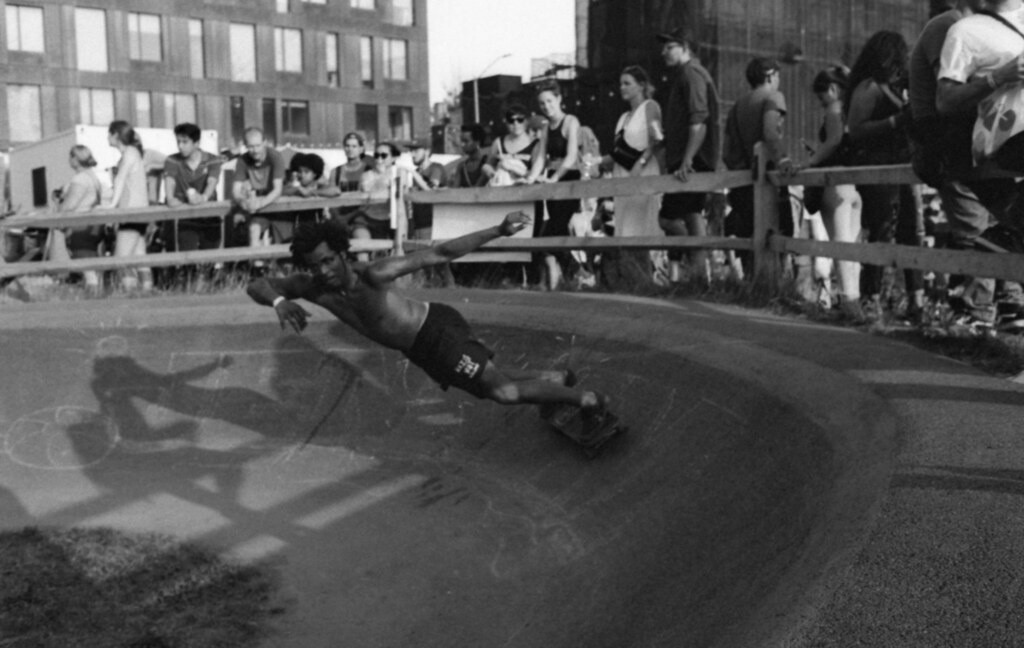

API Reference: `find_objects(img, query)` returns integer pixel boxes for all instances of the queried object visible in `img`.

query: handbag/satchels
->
[609,129,643,171]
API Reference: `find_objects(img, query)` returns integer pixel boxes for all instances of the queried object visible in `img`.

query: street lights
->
[473,53,514,125]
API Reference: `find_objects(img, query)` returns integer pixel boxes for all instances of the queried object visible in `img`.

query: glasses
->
[507,117,527,124]
[375,152,387,159]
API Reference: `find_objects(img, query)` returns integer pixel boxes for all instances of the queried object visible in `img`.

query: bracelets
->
[638,157,646,166]
[777,156,791,168]
[292,180,301,188]
[888,116,897,129]
[986,71,997,90]
[272,295,285,307]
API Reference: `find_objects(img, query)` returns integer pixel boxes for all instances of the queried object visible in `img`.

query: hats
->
[656,26,695,45]
[342,133,364,158]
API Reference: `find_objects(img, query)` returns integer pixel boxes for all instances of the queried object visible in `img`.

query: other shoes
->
[581,389,611,439]
[539,369,576,419]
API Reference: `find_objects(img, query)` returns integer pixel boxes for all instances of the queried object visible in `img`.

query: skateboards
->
[536,401,630,458]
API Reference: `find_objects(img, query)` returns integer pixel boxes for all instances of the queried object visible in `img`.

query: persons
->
[801,200,835,311]
[842,29,941,329]
[52,143,106,293]
[783,63,868,325]
[246,209,611,441]
[513,80,593,292]
[230,126,285,280]
[903,0,1024,337]
[271,153,341,243]
[91,121,154,293]
[648,26,721,289]
[579,64,666,286]
[722,56,799,283]
[447,103,544,290]
[164,123,224,291]
[327,131,454,289]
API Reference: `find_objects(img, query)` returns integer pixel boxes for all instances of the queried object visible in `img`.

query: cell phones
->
[801,137,815,154]
